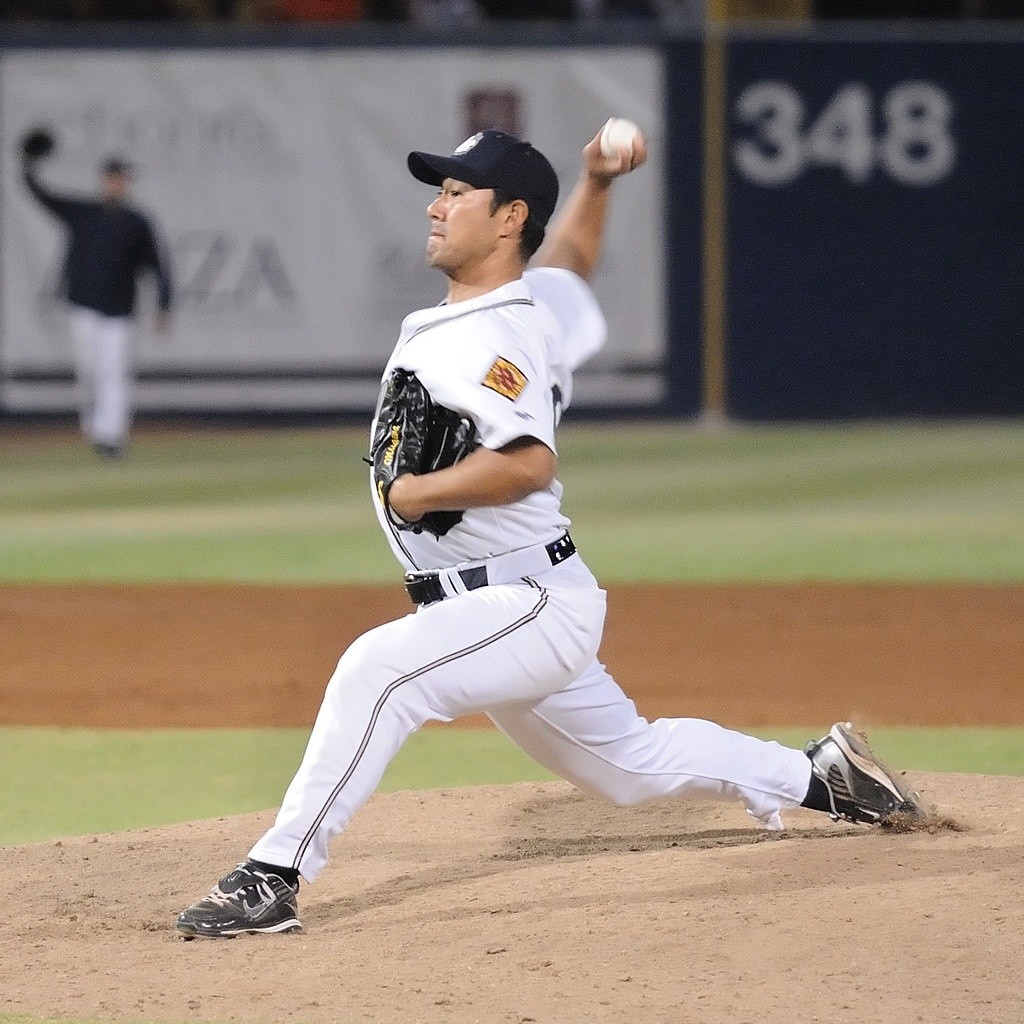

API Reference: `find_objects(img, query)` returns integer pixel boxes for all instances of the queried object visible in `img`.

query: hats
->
[406,129,560,224]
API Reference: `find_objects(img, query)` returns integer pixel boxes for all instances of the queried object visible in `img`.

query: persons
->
[186,1,367,48]
[176,118,929,942]
[20,129,171,454]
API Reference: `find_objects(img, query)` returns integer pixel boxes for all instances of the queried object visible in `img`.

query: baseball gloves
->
[368,366,480,537]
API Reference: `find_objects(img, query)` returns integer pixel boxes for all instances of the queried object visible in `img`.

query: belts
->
[404,529,575,603]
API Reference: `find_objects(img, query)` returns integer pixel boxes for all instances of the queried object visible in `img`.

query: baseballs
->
[600,119,641,161]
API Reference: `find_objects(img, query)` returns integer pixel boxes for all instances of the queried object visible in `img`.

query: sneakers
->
[176,862,303,936]
[803,721,928,829]
